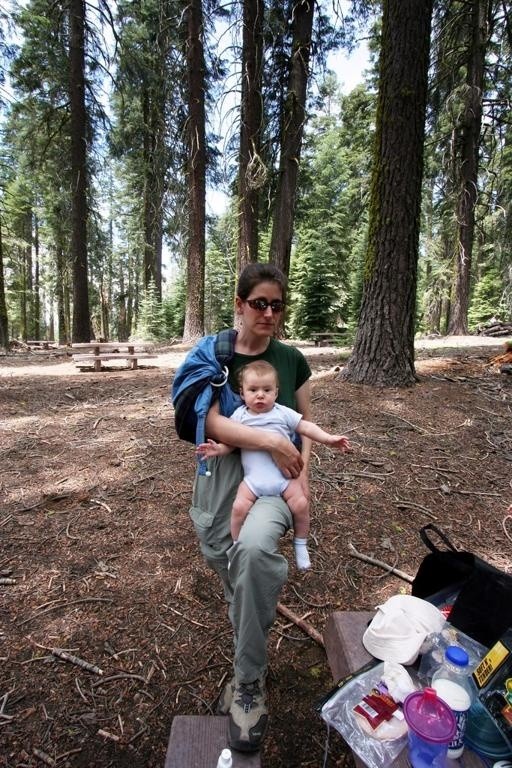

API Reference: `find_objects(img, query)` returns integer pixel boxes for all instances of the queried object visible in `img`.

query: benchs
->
[74,353,158,372]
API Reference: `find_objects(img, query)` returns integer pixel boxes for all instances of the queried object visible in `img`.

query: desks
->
[72,342,151,369]
[318,609,511,766]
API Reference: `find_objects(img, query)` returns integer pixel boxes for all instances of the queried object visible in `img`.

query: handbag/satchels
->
[410,524,511,649]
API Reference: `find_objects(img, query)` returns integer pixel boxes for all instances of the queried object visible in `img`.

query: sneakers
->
[216,675,234,715]
[228,666,270,752]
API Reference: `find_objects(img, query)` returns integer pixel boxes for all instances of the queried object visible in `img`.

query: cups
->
[405,688,456,768]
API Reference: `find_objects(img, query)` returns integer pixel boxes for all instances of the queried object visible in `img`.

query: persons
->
[174,260,315,753]
[195,357,350,571]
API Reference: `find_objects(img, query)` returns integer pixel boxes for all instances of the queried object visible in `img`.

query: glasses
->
[240,296,286,313]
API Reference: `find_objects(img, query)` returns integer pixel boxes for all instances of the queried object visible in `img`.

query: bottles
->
[429,648,469,759]
[216,749,232,768]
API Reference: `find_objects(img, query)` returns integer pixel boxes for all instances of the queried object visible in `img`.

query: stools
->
[165,715,266,767]
